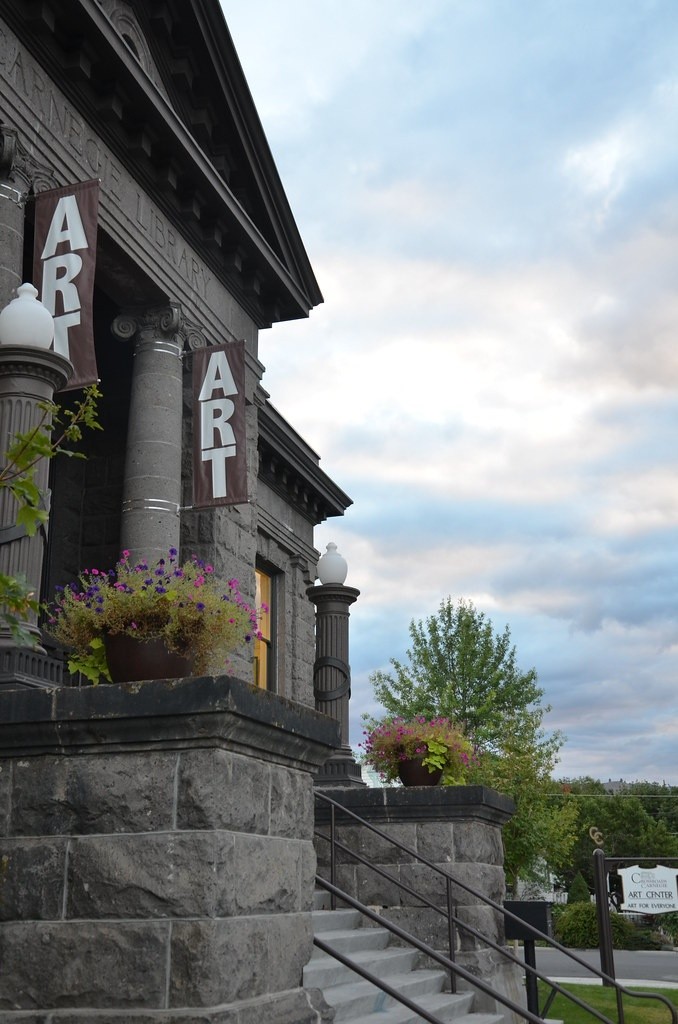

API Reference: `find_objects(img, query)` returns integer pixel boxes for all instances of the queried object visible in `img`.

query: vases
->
[99,618,193,682]
[397,759,445,786]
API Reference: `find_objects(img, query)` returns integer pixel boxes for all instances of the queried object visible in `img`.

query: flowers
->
[42,546,270,676]
[357,715,483,786]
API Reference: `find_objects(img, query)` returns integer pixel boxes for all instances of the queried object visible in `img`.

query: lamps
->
[0,283,55,349]
[317,543,348,585]
